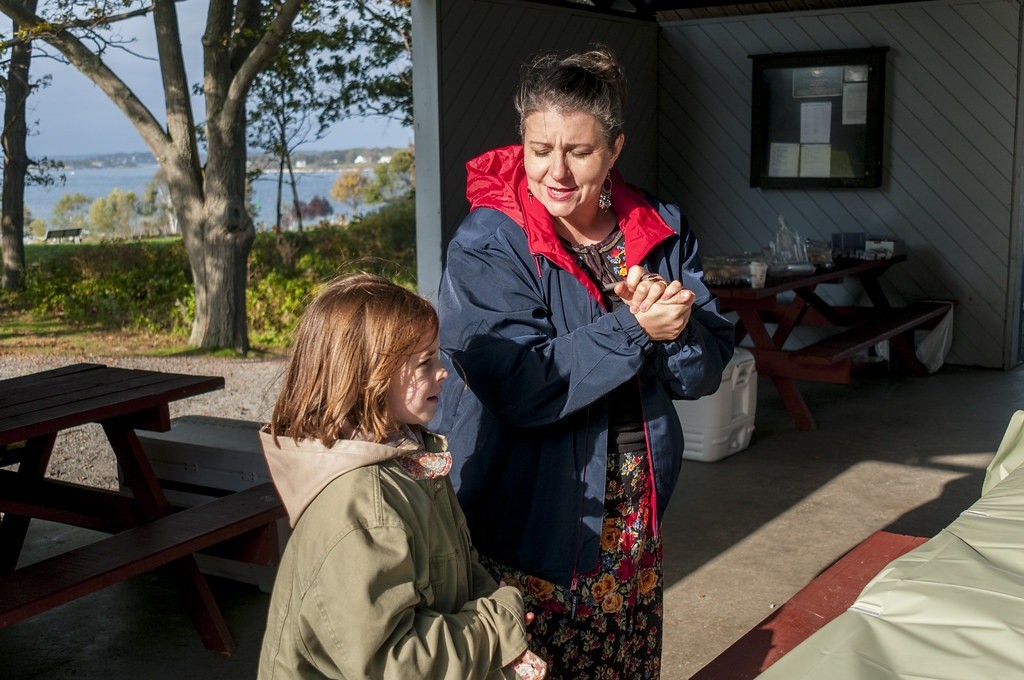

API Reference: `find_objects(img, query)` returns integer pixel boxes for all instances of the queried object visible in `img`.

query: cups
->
[749,261,768,290]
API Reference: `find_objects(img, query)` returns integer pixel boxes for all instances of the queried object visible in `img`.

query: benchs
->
[788,299,960,384]
[687,531,931,680]
[39,227,83,244]
[0,482,288,628]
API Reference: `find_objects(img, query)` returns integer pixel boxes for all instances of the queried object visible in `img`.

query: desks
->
[750,408,1024,680]
[1,362,236,655]
[709,253,908,432]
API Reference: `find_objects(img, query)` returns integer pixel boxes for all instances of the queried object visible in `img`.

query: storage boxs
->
[671,348,757,463]
[118,414,298,590]
[865,240,904,258]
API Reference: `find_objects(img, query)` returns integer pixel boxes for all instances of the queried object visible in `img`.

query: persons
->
[258,272,536,680]
[435,46,735,680]
